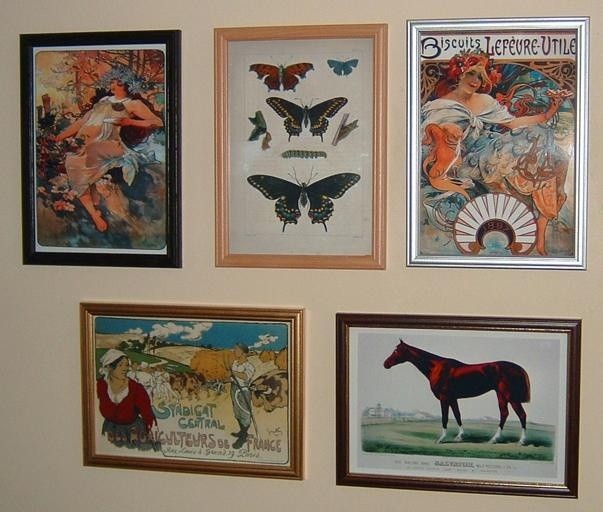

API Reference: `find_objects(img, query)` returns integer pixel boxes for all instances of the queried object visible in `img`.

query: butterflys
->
[248,56,314,93]
[265,97,347,142]
[327,58,357,78]
[246,166,360,232]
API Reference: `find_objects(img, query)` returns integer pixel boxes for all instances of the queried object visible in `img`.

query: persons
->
[46,64,162,234]
[94,348,157,451]
[225,337,257,451]
[420,48,575,256]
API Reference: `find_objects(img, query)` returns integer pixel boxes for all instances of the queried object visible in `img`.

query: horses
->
[383,339,530,445]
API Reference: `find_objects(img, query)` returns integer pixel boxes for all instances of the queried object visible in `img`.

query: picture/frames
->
[401,15,591,273]
[77,298,306,483]
[333,310,582,500]
[209,22,389,273]
[16,28,182,270]
